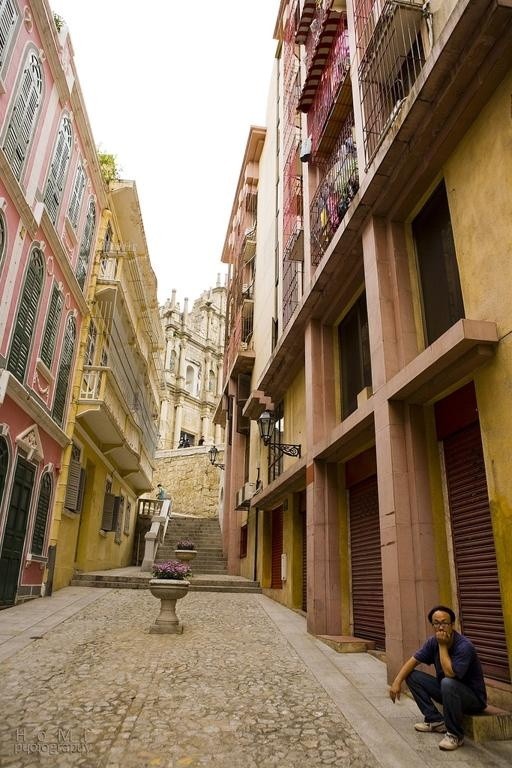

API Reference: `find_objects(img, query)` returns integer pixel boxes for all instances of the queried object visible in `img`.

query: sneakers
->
[414,720,447,733]
[438,730,464,750]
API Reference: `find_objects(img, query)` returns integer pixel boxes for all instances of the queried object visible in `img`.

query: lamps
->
[257,408,303,458]
[208,446,224,472]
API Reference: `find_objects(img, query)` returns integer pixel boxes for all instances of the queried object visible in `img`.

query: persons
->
[155,484,165,512]
[389,605,488,751]
[177,435,206,449]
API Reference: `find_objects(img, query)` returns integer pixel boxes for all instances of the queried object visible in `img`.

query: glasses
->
[432,622,451,627]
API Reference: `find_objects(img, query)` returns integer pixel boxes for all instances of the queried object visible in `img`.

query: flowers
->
[153,561,190,579]
[175,537,195,549]
